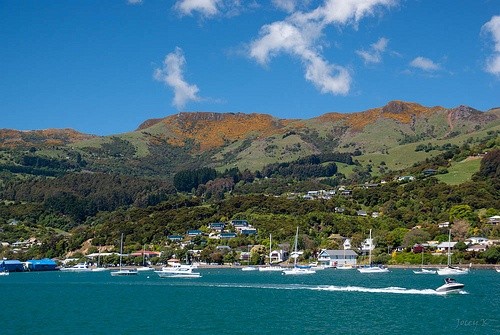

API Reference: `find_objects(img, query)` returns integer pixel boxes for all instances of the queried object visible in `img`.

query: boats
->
[435,282,464,291]
[154,266,201,278]
[413,268,437,274]
[60,264,91,271]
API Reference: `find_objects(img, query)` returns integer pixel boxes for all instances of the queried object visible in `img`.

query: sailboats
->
[336,242,356,269]
[241,243,259,271]
[111,233,137,276]
[282,225,316,274]
[357,228,390,273]
[258,233,289,272]
[437,229,469,275]
